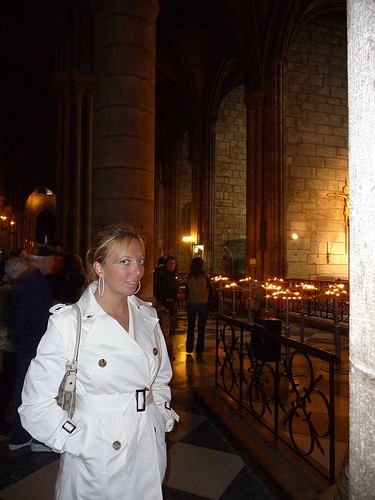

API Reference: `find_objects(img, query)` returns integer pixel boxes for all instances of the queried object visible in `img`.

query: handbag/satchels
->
[53,361,81,419]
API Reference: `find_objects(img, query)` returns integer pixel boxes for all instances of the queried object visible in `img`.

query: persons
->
[185,257,215,357]
[18,224,179,500]
[156,256,178,361]
[5,247,98,303]
[5,246,57,453]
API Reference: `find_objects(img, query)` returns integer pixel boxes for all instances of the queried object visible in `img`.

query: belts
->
[74,388,154,412]
